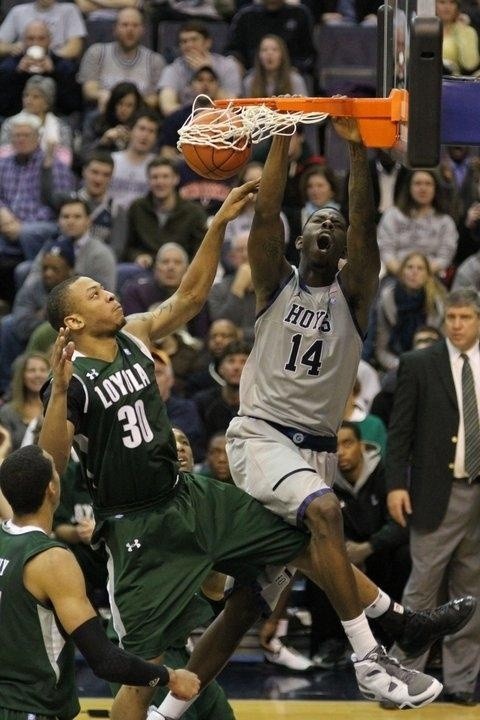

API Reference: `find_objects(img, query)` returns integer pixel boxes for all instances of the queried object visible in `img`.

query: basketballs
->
[179,107,252,181]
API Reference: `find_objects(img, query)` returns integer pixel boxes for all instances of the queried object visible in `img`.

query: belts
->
[453,474,480,486]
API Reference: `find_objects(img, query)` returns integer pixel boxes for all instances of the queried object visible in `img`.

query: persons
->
[1,1,479,719]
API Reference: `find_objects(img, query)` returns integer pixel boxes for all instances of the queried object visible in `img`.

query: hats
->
[47,231,76,269]
[153,347,173,370]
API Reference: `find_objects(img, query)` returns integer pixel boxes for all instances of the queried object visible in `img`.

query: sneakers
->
[264,637,317,672]
[393,594,476,658]
[313,640,346,668]
[452,689,475,705]
[348,651,441,708]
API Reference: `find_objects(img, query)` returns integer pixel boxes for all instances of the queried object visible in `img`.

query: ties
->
[456,352,479,478]
